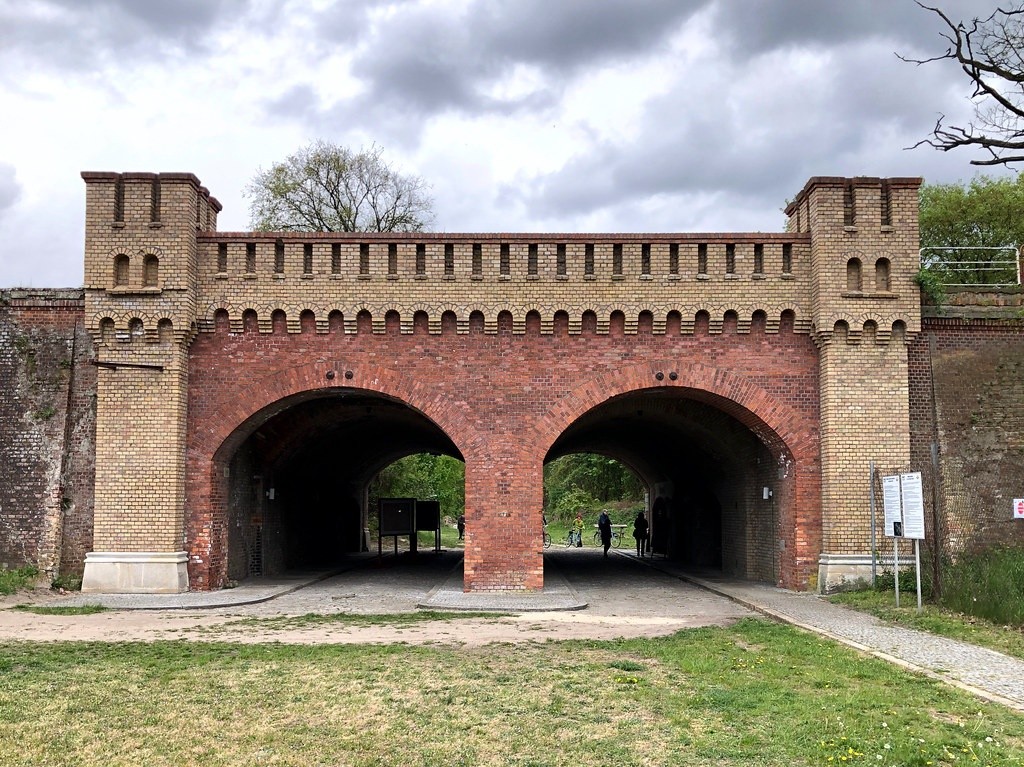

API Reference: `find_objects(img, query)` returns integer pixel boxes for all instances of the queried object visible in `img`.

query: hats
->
[576,513,582,518]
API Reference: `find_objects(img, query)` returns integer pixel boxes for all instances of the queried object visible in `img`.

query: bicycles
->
[565,528,581,548]
[461,529,465,540]
[542,525,551,549]
[594,528,621,549]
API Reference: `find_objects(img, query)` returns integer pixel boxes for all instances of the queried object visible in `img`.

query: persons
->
[601,520,612,555]
[598,509,613,531]
[573,513,585,547]
[633,513,648,554]
[458,513,465,539]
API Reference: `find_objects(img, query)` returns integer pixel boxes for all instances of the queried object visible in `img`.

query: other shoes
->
[604,554,608,558]
